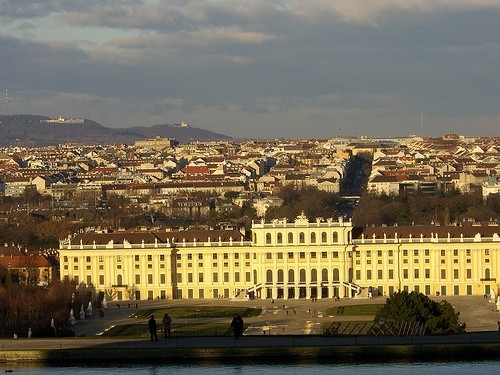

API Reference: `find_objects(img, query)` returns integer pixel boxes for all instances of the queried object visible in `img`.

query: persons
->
[162,312,174,338]
[38,282,146,342]
[260,282,395,336]
[227,312,245,342]
[146,315,158,341]
[147,286,242,310]
